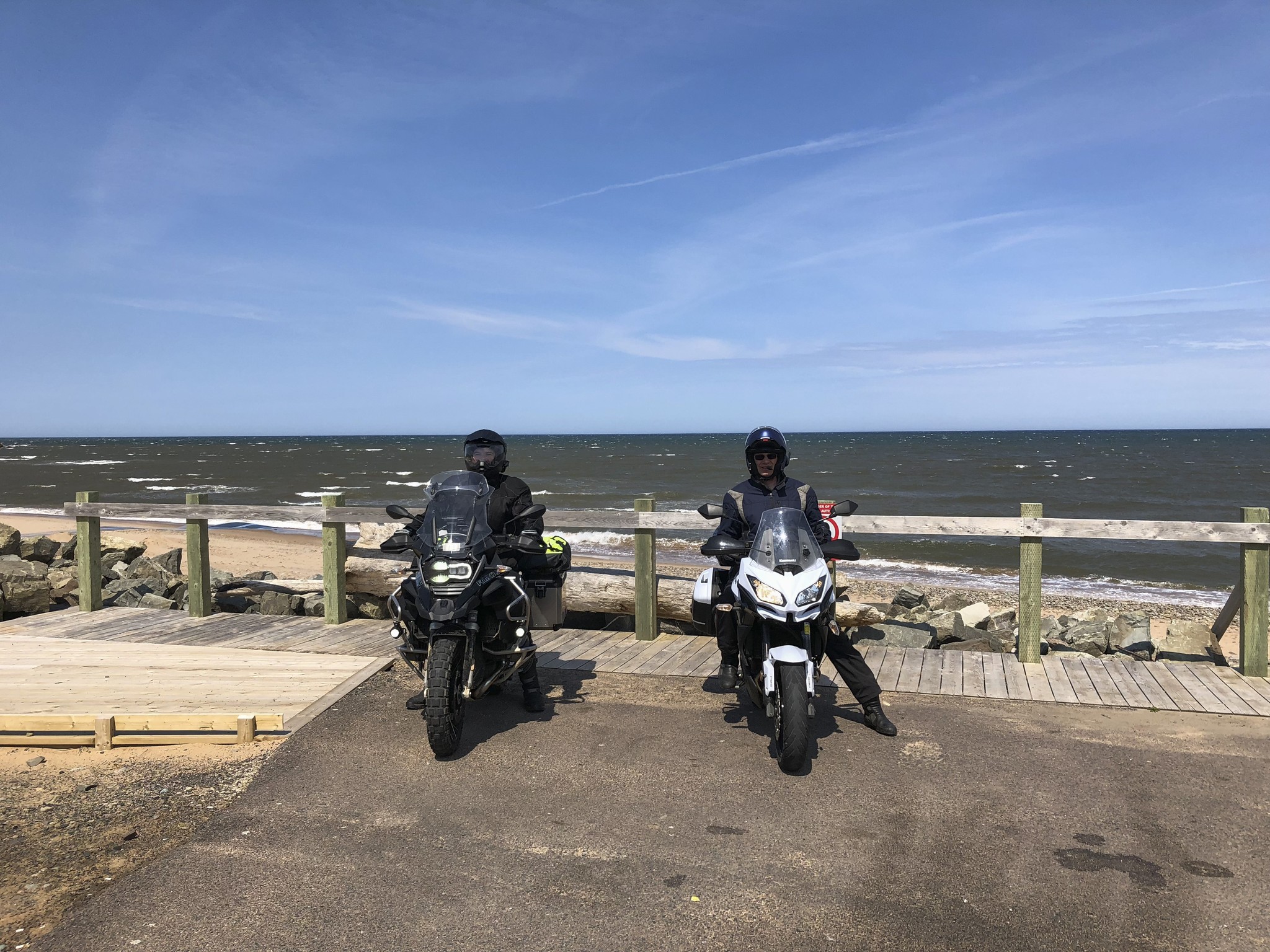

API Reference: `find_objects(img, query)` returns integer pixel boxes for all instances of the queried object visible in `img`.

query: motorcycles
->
[691,499,861,772]
[380,469,565,758]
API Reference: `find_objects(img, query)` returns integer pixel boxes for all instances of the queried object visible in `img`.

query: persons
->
[713,426,897,736]
[380,429,545,712]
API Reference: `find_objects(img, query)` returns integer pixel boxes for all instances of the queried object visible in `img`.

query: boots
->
[521,666,546,712]
[406,688,425,710]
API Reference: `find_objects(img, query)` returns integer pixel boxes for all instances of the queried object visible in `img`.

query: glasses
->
[754,453,777,461]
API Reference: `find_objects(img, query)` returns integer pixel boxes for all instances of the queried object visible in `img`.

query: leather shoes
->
[863,696,897,736]
[719,655,738,691]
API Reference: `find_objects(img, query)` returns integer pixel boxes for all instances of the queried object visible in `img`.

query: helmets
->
[464,429,510,478]
[744,425,791,473]
